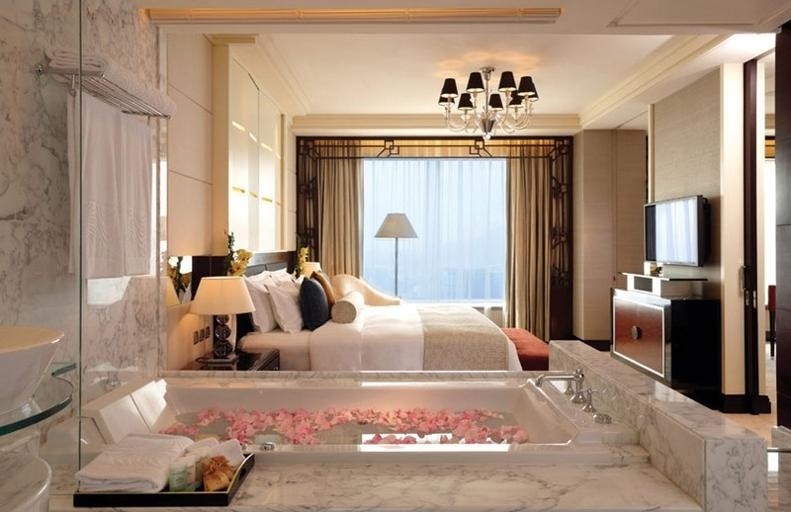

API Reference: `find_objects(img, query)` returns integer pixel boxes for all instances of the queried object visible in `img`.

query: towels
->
[44,45,176,118]
[75,431,245,493]
[67,89,153,281]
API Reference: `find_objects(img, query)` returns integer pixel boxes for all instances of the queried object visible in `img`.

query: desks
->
[610,272,720,390]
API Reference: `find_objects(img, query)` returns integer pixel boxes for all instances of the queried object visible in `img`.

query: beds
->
[237,261,523,370]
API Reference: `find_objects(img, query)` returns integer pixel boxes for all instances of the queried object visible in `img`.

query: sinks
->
[0,326,64,412]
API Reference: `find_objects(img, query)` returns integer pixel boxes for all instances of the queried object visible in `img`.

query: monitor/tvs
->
[643,194,713,268]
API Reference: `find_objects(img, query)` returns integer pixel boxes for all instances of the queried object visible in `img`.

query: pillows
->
[242,267,364,334]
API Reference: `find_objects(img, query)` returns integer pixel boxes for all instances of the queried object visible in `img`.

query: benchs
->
[501,327,549,370]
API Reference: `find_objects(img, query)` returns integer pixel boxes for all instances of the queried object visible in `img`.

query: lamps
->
[374,213,418,296]
[438,67,538,141]
[187,276,257,363]
[304,261,322,278]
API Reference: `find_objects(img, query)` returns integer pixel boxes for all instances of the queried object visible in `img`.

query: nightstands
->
[180,349,280,371]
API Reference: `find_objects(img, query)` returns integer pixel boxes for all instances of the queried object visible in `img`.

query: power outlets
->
[193,326,210,345]
